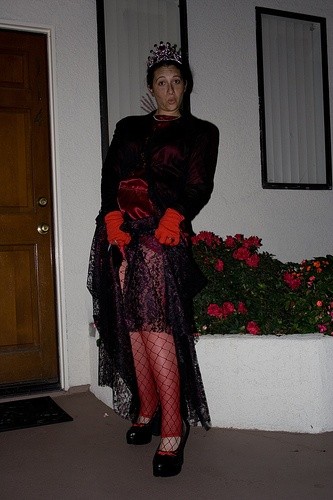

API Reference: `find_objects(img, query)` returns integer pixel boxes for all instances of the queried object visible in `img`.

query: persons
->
[101,57,222,478]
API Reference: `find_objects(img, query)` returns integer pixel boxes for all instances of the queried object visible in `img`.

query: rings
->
[166,237,175,242]
[113,239,117,244]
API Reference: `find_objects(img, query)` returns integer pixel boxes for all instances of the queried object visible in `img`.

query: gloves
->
[104,210,132,257]
[154,208,185,246]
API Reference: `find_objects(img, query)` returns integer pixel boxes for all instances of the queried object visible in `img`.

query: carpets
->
[0,396,73,432]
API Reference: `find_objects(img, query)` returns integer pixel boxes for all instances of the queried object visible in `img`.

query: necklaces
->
[151,114,183,122]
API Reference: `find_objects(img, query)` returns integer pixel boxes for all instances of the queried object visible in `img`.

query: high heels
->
[153,418,190,477]
[126,405,162,445]
